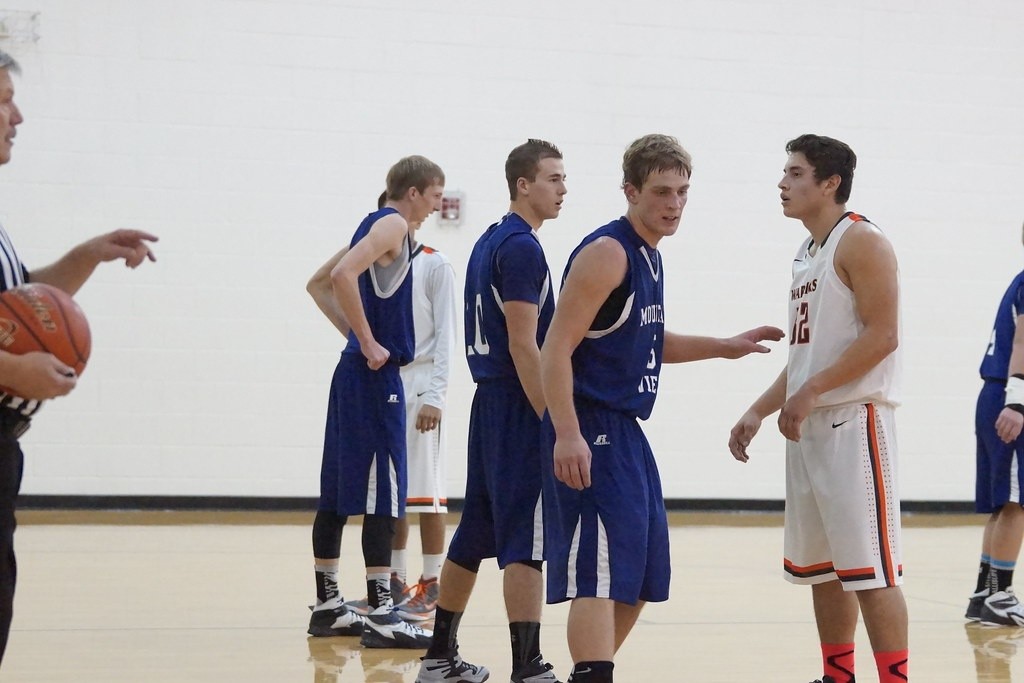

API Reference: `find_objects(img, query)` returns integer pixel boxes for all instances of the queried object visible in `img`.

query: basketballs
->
[1,282,92,397]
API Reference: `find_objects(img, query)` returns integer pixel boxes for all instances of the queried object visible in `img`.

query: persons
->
[966,223,1024,627]
[307,155,445,647]
[729,135,907,683]
[539,133,785,683]
[416,140,567,682]
[0,50,159,667]
[343,190,456,622]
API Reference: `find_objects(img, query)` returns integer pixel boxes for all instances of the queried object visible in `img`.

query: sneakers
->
[964,588,990,621]
[965,620,1024,653]
[509,654,564,683]
[307,596,366,636]
[415,645,490,683]
[307,636,365,674]
[361,648,428,676]
[396,574,439,621]
[981,585,1024,628]
[390,572,411,607]
[359,598,435,649]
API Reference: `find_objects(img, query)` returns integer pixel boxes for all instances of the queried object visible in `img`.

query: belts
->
[0,412,30,439]
